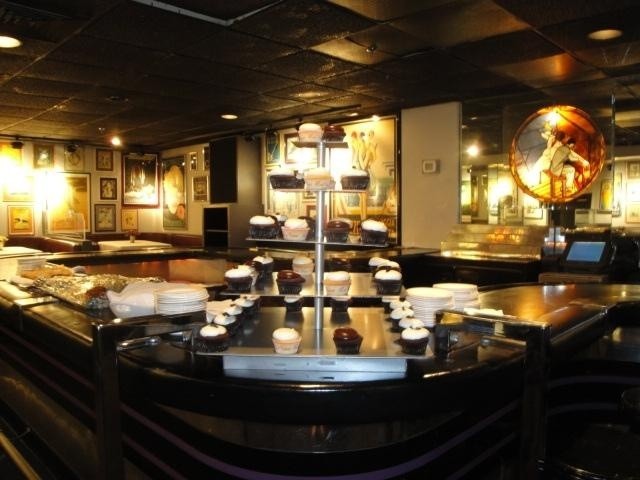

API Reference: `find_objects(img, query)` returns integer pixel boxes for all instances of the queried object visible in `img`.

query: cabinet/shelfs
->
[223,139,408,371]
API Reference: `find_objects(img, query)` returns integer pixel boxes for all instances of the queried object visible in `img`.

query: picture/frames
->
[626,161,639,178]
[1,142,210,244]
[625,201,640,224]
[265,132,395,222]
[599,180,614,213]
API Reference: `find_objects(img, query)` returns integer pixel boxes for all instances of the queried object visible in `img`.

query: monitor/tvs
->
[560,232,614,272]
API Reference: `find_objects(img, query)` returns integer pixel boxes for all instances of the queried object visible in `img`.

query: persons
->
[162,180,185,228]
[548,137,590,194]
[349,128,382,172]
[522,127,566,192]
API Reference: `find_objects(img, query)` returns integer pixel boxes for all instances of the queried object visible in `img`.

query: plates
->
[405,283,480,329]
[15,257,46,276]
[153,284,210,315]
